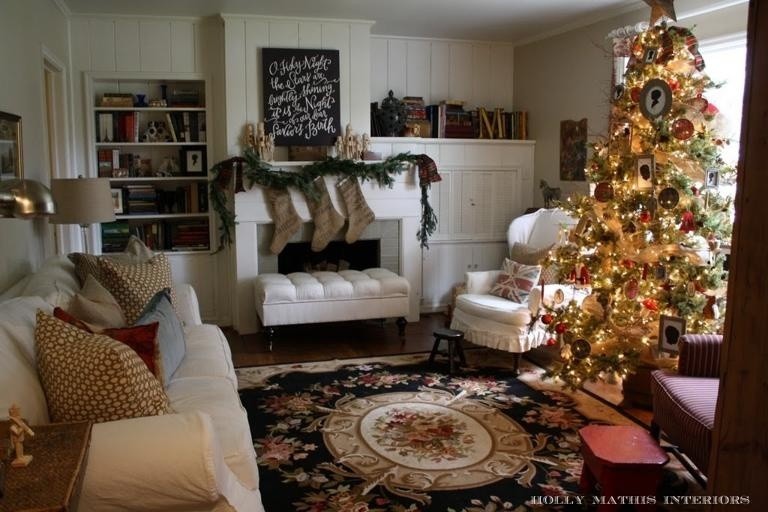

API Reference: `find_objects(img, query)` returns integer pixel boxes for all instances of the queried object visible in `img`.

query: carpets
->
[233,345,711,512]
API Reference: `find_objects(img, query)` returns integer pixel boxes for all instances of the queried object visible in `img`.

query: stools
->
[576,424,670,512]
[428,330,466,374]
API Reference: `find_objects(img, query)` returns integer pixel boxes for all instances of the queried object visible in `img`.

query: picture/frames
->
[181,145,207,177]
[633,154,656,191]
[612,85,624,101]
[658,315,685,355]
[0,110,24,218]
[704,169,719,189]
[642,47,658,65]
[639,78,672,120]
[714,245,732,282]
[111,186,125,215]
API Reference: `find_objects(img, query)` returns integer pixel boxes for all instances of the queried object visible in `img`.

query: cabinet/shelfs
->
[367,141,535,315]
[81,67,233,328]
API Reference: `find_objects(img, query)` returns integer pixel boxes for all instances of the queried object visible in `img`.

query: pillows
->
[488,257,541,303]
[510,241,557,286]
[0,234,188,423]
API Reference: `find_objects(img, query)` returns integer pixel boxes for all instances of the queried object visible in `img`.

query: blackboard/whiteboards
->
[263,48,340,146]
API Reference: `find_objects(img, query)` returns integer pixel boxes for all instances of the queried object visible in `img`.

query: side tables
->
[0,419,97,512]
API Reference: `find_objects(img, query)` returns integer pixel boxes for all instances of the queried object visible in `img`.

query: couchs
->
[0,250,266,512]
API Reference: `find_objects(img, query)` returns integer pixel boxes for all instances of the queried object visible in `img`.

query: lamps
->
[0,178,57,219]
[48,173,117,253]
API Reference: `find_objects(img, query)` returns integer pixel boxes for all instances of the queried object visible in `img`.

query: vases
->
[133,95,147,107]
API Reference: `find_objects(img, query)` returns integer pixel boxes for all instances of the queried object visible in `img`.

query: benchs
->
[252,267,410,353]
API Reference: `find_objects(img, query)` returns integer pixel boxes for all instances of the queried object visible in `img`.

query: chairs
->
[648,333,724,478]
[449,207,593,375]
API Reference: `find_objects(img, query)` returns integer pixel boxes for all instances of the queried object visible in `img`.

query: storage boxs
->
[288,145,328,161]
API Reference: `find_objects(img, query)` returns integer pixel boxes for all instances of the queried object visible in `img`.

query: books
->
[425,95,528,140]
[95,84,210,254]
